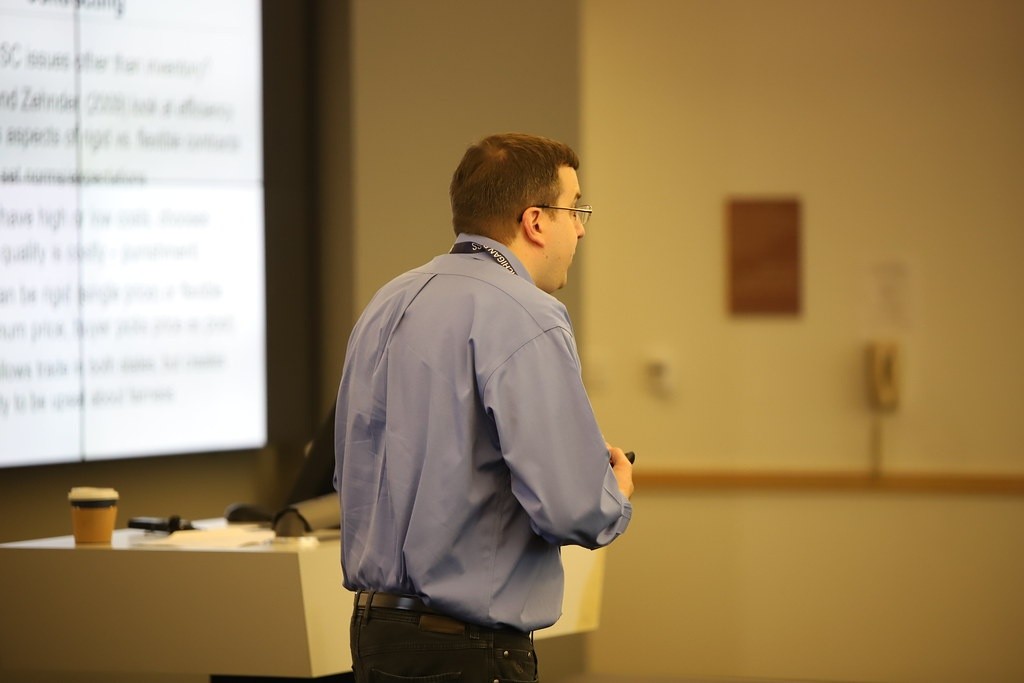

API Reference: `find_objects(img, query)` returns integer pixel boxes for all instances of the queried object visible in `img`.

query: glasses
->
[518,201,594,228]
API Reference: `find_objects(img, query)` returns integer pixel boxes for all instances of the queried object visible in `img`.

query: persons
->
[336,132,637,683]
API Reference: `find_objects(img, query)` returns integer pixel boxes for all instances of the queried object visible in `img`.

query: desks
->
[0,511,604,682]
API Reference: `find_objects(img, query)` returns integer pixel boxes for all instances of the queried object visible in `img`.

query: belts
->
[350,589,533,635]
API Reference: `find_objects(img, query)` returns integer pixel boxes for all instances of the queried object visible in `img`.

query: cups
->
[68,487,120,543]
